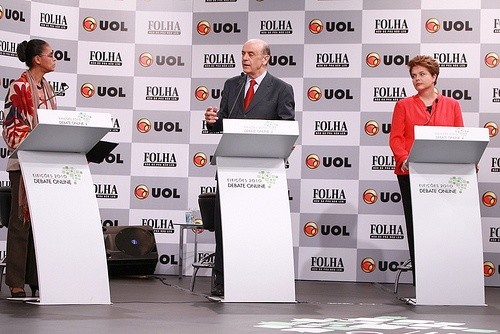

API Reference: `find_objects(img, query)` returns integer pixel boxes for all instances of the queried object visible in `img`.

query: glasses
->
[40,49,54,58]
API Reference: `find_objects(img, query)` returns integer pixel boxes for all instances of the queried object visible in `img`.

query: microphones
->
[228,72,246,118]
[38,82,69,109]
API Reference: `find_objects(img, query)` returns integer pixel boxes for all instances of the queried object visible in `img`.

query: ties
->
[244,80,256,112]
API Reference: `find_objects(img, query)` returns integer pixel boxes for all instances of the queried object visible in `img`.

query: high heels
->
[29,285,39,296]
[9,286,26,298]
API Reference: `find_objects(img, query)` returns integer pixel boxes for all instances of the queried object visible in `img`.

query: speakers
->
[103,225,157,277]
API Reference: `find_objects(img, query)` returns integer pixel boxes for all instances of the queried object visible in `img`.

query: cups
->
[185,211,193,223]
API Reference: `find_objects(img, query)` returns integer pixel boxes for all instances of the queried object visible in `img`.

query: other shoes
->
[211,283,224,296]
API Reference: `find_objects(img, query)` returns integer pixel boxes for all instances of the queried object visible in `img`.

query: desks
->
[172,223,204,280]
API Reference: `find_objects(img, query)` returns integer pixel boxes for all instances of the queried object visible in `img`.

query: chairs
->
[0,186,12,296]
[190,192,217,297]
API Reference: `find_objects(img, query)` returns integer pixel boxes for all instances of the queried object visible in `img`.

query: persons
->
[2,39,58,298]
[204,38,295,297]
[389,55,463,286]
